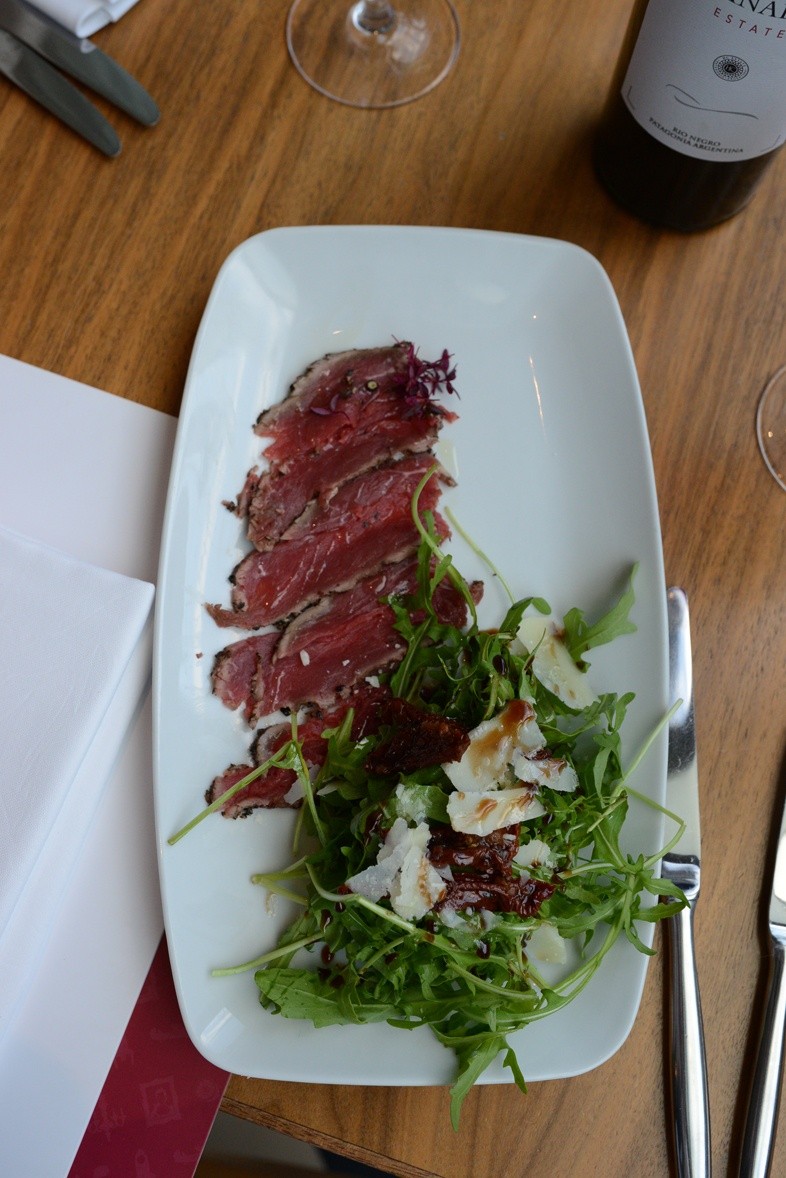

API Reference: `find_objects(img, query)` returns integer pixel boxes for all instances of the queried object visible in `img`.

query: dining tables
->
[0,0,786,1178]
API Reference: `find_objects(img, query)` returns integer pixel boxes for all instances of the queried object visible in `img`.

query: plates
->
[147,223,674,1090]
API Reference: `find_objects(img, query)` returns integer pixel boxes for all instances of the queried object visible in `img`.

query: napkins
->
[0,536,154,934]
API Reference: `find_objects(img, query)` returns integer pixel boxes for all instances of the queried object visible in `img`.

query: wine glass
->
[287,0,460,109]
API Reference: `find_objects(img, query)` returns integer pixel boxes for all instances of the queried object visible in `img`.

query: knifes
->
[664,584,716,1178]
[0,0,163,160]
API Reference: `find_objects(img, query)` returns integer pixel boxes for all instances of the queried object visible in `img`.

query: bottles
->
[586,0,785,234]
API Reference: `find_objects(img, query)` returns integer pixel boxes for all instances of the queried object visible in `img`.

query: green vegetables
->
[162,461,695,1130]
